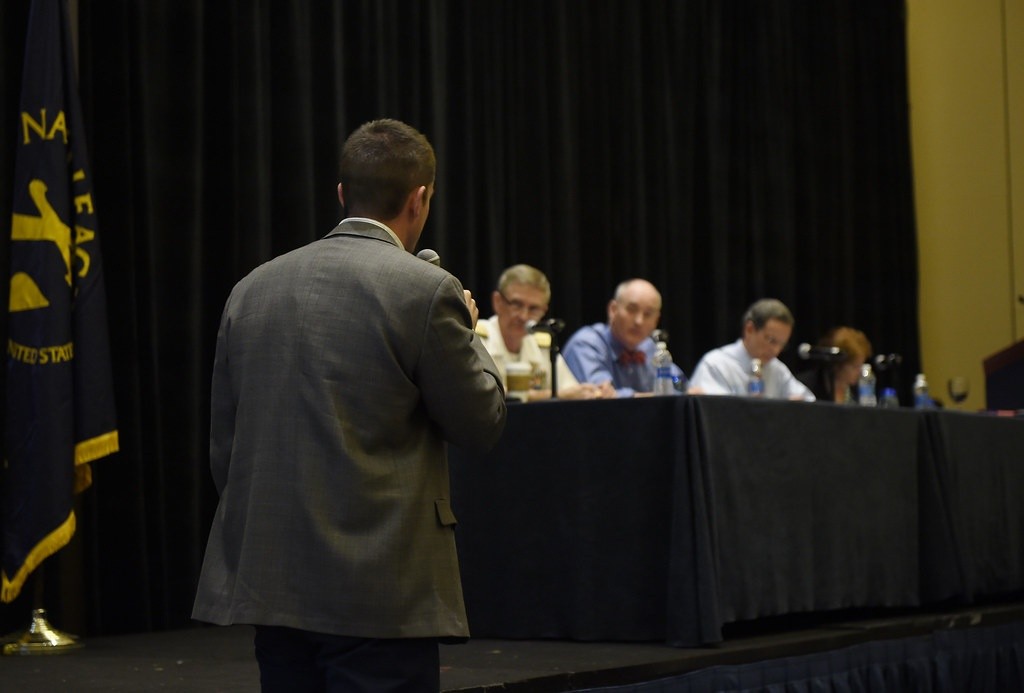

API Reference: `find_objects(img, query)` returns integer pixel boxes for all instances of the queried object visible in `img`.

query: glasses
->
[499,289,547,316]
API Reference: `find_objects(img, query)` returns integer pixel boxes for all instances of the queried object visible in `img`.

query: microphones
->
[417,249,440,267]
[799,342,847,362]
[876,354,901,368]
[652,329,666,349]
[524,320,564,334]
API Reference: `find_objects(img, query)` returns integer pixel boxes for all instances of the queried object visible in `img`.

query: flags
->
[0,0,122,606]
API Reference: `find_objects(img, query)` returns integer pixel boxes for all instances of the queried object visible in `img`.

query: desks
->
[448,388,967,649]
[920,409,1024,610]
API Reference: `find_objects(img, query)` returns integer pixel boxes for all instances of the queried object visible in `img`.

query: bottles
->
[747,358,764,397]
[653,343,674,395]
[857,364,876,407]
[879,388,898,408]
[914,374,930,410]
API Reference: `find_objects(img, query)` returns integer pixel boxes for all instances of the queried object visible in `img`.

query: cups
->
[506,362,533,403]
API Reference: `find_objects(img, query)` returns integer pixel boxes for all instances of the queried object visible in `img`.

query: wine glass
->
[949,376,969,411]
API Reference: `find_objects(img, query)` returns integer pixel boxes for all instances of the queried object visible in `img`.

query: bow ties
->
[619,350,646,367]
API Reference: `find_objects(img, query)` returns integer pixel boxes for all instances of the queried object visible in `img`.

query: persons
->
[797,328,873,403]
[562,279,703,395]
[468,266,616,397]
[689,300,818,402]
[187,119,508,693]
